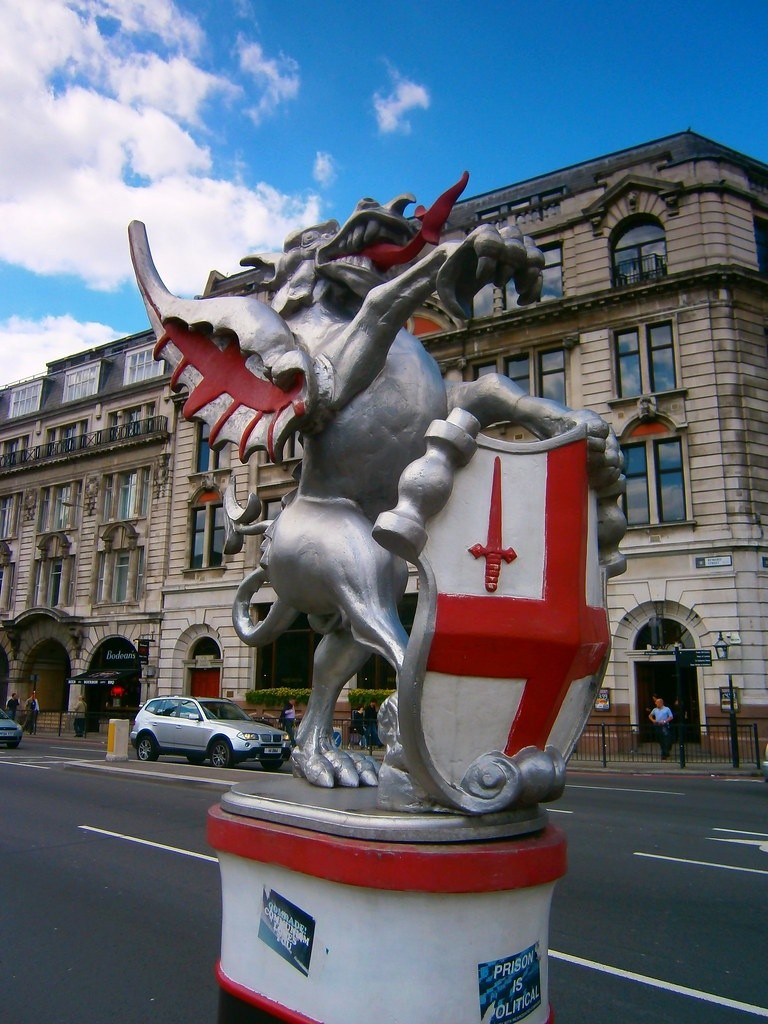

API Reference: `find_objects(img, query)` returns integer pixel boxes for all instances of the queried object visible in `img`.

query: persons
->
[23,691,40,735]
[72,694,87,738]
[282,698,296,748]
[347,706,364,750]
[364,698,377,749]
[647,699,673,759]
[645,693,688,742]
[5,692,20,721]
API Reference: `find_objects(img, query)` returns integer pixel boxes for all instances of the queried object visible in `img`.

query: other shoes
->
[661,752,670,760]
[73,734,83,737]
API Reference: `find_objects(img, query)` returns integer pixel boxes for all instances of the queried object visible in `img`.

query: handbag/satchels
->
[350,730,362,743]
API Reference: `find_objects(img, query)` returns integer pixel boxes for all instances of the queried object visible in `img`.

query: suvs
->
[130,695,293,770]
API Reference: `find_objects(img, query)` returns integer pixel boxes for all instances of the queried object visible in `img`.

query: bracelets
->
[664,720,666,725]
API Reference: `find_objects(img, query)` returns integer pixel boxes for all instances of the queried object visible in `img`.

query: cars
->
[0,708,23,749]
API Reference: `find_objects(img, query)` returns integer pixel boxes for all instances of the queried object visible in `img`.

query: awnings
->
[65,669,139,685]
